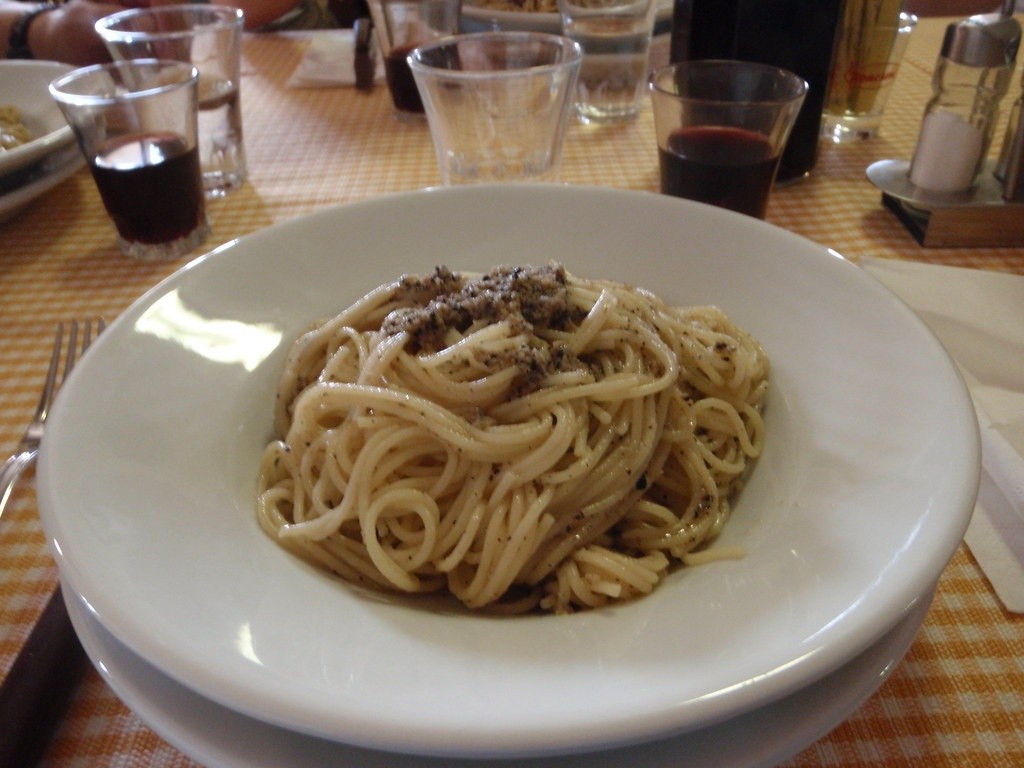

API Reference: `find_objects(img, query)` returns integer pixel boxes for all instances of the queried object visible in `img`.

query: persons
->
[0,0,154,80]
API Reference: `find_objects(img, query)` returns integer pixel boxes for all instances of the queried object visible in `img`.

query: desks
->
[0,15,1024,768]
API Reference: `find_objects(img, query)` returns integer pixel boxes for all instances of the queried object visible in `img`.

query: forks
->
[0,319,106,517]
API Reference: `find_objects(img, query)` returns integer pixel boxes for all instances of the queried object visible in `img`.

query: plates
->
[457,3,671,62]
[39,179,981,765]
[0,60,113,216]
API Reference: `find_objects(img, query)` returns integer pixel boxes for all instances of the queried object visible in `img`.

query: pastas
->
[255,262,772,613]
[473,0,604,13]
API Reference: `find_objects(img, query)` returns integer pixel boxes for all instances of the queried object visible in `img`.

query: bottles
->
[908,12,1022,193]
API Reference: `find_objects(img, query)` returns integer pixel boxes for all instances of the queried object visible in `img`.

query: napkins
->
[858,258,1024,616]
[284,32,385,90]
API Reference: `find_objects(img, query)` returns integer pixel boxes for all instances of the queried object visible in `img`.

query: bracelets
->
[5,3,53,59]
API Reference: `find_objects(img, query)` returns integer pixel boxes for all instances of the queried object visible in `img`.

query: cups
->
[668,0,849,187]
[366,0,463,122]
[648,60,809,222]
[96,4,247,195]
[48,58,210,261]
[405,31,583,187]
[556,0,657,125]
[821,0,918,144]
